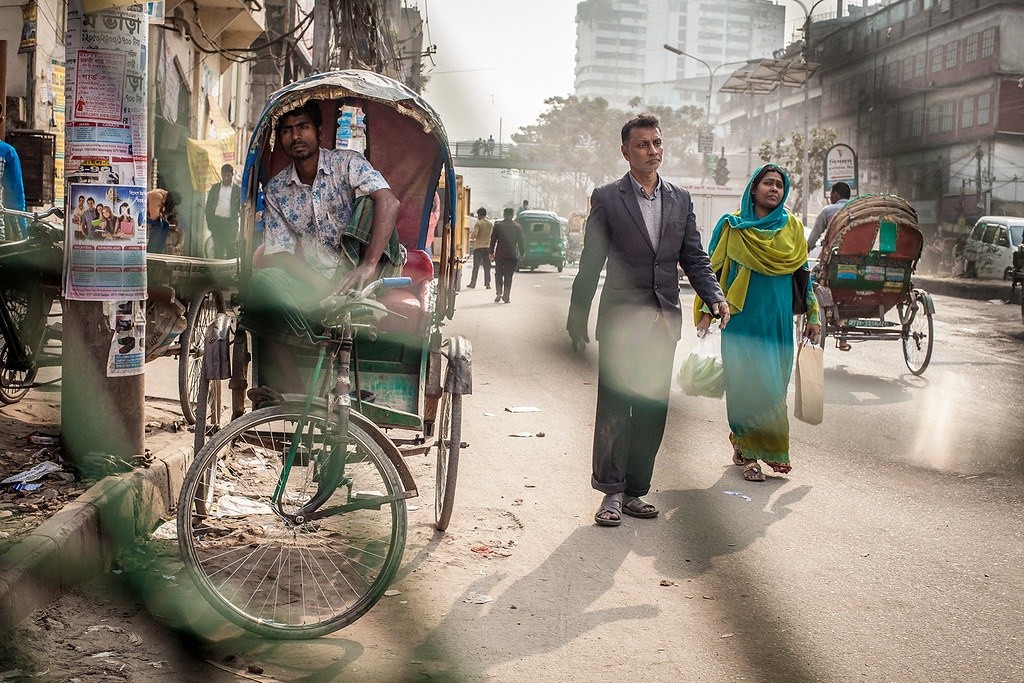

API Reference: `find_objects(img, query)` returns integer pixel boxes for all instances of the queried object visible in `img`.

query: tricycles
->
[0,203,241,425]
[563,211,591,266]
[794,193,935,376]
[175,68,474,641]
[459,237,499,268]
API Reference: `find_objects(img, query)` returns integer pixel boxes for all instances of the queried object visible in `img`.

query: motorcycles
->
[516,210,567,274]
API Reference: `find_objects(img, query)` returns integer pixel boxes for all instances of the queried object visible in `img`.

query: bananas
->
[676,353,725,398]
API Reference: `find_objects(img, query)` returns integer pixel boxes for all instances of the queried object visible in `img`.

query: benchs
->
[365,249,435,339]
[817,252,919,298]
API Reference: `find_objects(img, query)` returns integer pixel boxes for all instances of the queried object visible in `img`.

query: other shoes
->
[467,285,475,288]
[350,390,375,402]
[495,296,501,301]
[486,287,491,289]
[246,386,285,405]
[502,297,508,303]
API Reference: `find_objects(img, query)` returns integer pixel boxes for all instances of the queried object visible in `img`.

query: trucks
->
[962,215,1024,284]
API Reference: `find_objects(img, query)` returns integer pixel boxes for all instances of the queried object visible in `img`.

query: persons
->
[0,103,27,333]
[205,165,244,261]
[472,136,531,304]
[246,99,400,404]
[71,196,136,242]
[147,188,185,256]
[805,182,852,350]
[568,115,731,525]
[693,162,824,481]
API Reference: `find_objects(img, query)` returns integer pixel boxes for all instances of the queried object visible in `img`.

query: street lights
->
[663,44,764,186]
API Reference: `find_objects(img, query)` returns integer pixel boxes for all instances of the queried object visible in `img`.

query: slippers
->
[622,495,659,517]
[595,494,623,526]
[729,433,745,464]
[744,460,765,481]
[840,344,851,351]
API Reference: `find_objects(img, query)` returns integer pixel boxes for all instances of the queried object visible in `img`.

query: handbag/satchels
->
[794,336,823,425]
[678,329,725,399]
